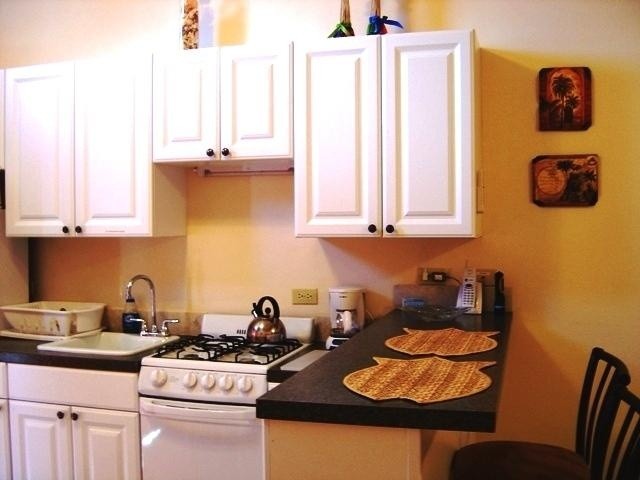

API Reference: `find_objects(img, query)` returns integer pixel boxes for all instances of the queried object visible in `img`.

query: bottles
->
[122,300,141,333]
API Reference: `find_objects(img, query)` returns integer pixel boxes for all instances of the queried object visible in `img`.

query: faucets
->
[125,274,180,336]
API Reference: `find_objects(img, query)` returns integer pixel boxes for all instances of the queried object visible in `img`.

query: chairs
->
[598,390,640,478]
[453,347,631,480]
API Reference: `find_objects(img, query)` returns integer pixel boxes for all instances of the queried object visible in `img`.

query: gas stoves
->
[137,313,314,405]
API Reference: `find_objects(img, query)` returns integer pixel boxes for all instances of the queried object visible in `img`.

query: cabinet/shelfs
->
[138,397,260,479]
[1,364,142,480]
[293,31,478,237]
[5,55,187,239]
[152,43,294,172]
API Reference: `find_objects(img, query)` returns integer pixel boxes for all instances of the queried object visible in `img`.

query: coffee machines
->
[326,288,366,351]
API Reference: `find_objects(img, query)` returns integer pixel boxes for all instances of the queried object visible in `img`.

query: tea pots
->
[246,295,288,345]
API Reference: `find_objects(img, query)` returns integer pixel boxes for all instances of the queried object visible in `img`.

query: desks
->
[256,309,511,479]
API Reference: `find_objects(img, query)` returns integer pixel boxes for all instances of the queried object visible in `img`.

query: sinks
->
[36,327,182,357]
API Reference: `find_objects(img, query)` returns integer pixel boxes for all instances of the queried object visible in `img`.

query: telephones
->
[456,259,482,314]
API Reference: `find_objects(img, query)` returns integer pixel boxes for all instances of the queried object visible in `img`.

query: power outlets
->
[293,287,319,304]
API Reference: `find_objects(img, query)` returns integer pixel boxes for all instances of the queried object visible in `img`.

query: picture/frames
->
[537,68,592,131]
[529,154,601,208]
